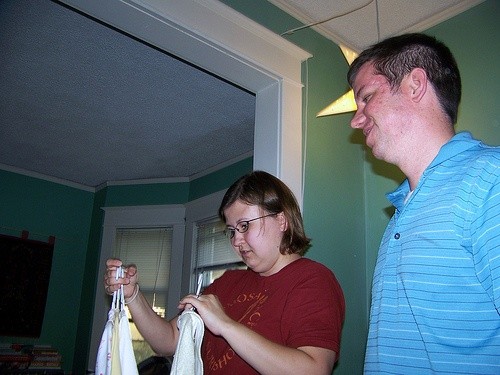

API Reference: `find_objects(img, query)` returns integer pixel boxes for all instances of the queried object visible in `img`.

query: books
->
[0,344,65,370]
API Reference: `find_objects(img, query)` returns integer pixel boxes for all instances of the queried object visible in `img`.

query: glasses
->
[225,212,278,239]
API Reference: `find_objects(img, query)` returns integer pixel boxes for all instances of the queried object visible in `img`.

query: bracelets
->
[125,283,140,305]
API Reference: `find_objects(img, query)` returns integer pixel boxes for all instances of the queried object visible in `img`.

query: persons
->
[104,170,345,375]
[346,33,500,375]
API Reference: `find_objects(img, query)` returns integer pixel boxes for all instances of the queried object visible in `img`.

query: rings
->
[196,291,203,299]
